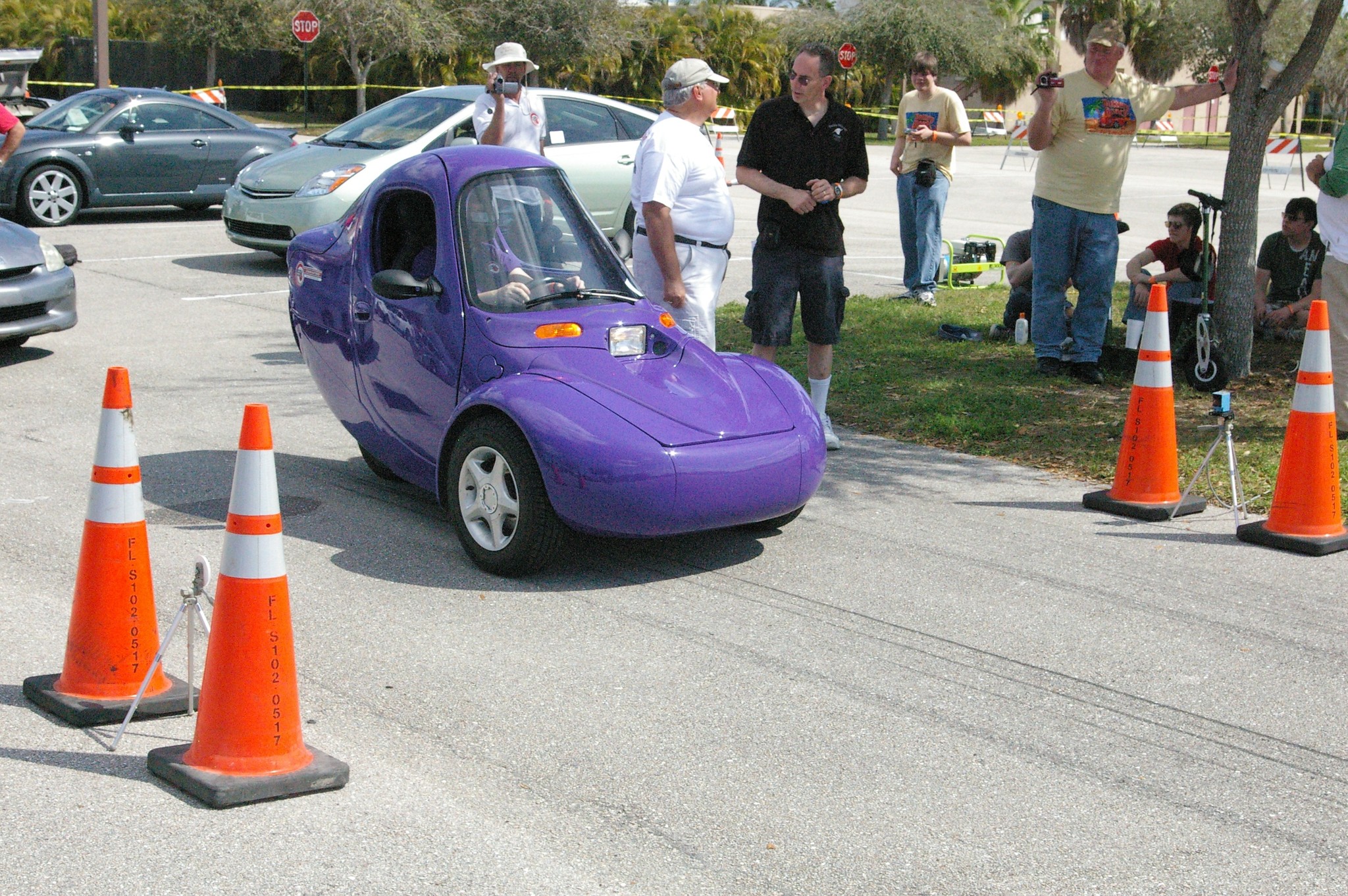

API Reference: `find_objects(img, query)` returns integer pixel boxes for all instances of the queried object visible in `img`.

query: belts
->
[636,226,728,250]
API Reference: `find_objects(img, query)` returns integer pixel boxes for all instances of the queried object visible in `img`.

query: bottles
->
[1015,313,1029,346]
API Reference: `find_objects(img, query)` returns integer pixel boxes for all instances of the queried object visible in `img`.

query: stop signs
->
[291,10,320,43]
[1208,66,1220,84]
[838,43,858,69]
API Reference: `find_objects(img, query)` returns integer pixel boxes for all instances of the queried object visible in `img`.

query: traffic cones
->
[712,128,725,167]
[1236,300,1348,557]
[145,403,349,810]
[23,364,202,728]
[1083,283,1208,522]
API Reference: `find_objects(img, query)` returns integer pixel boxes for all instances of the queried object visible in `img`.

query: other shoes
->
[1110,349,1137,374]
[1336,429,1348,441]
[1284,328,1305,342]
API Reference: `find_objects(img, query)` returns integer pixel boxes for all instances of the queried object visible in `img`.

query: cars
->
[223,85,659,263]
[0,217,78,361]
[0,50,299,227]
[287,148,824,577]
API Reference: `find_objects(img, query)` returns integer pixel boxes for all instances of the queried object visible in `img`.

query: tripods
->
[1165,411,1248,531]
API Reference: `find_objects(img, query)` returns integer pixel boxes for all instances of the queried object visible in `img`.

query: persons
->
[630,58,735,353]
[1028,19,1238,385]
[988,229,1075,341]
[410,183,586,307]
[1109,203,1217,349]
[1306,120,1348,440]
[890,52,972,307]
[1253,197,1326,345]
[472,42,554,268]
[736,41,870,450]
[0,102,26,168]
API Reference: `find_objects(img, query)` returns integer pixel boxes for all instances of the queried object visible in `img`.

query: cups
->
[1125,319,1143,350]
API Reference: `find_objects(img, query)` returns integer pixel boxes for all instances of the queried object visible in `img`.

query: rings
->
[667,301,673,304]
[823,191,826,195]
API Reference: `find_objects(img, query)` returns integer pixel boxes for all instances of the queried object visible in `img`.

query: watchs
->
[831,183,840,197]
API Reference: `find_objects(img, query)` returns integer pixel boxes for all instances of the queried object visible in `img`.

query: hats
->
[938,324,982,341]
[482,42,539,75]
[662,57,730,89]
[1085,21,1126,47]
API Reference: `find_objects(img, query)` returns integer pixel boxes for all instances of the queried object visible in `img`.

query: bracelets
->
[0,160,3,165]
[550,282,556,294]
[931,130,937,142]
[1218,80,1227,96]
[1286,304,1294,316]
[834,182,844,199]
[1150,276,1157,284]
[543,199,552,204]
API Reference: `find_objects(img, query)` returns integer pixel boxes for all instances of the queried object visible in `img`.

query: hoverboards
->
[1102,189,1230,391]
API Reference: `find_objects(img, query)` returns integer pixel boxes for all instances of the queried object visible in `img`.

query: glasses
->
[788,70,828,86]
[1166,221,1194,229]
[1282,211,1310,223]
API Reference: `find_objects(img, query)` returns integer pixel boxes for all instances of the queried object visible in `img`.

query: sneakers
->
[818,414,840,450]
[1035,356,1062,377]
[917,288,937,307]
[1069,359,1104,384]
[989,324,1014,340]
[896,290,919,299]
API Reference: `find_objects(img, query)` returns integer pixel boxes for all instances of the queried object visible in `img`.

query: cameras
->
[904,128,918,140]
[493,73,519,95]
[1039,72,1064,88]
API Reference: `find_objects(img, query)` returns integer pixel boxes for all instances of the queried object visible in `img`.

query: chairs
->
[457,117,476,139]
[389,200,438,274]
